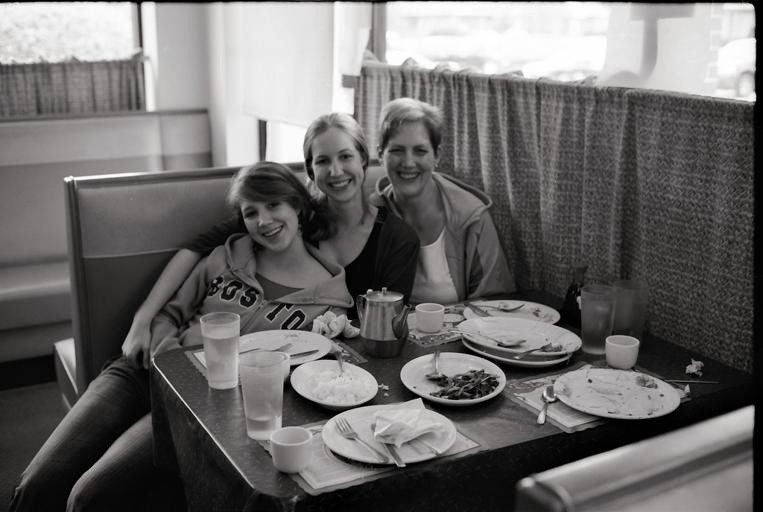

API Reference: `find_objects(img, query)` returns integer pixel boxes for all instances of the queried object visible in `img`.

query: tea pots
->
[355,286,413,359]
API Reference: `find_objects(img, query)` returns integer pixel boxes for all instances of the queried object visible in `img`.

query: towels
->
[372,398,447,453]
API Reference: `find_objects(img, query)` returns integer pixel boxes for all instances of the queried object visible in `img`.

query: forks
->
[332,417,388,464]
[471,302,525,312]
[239,343,294,353]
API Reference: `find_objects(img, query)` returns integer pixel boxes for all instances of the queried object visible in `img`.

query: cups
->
[581,285,617,356]
[414,302,444,333]
[199,310,240,390]
[613,277,650,343]
[604,334,640,371]
[239,350,286,441]
[271,426,314,473]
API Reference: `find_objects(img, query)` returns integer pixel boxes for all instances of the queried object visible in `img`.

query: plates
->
[457,316,583,355]
[238,329,331,366]
[290,359,379,406]
[553,368,682,420]
[321,404,457,465]
[461,336,573,366]
[399,352,507,407]
[463,299,561,325]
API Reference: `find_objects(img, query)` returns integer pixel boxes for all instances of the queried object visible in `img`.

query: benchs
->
[2,108,211,362]
[514,412,726,509]
[52,157,385,412]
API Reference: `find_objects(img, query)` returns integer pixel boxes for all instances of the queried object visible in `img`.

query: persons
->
[122,113,422,369]
[12,159,356,510]
[365,98,520,300]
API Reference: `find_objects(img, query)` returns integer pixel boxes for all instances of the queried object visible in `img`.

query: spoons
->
[536,385,557,425]
[448,329,526,347]
[290,348,320,358]
[426,349,446,380]
[513,342,564,359]
